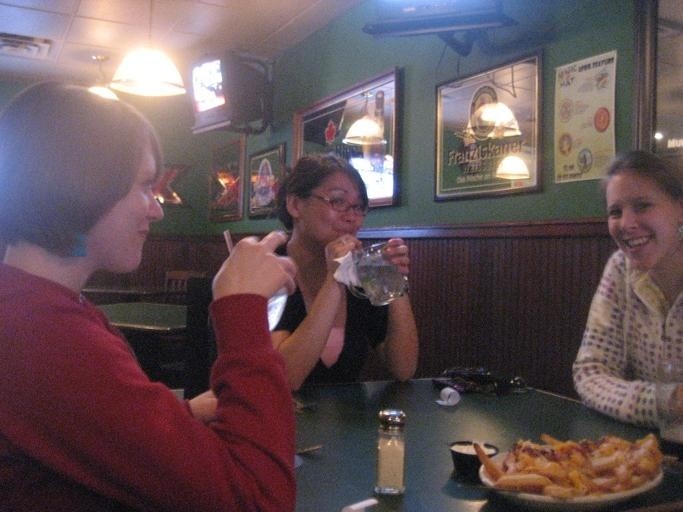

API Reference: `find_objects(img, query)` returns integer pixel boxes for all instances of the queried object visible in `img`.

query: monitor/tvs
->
[370,0,502,30]
[187,49,266,131]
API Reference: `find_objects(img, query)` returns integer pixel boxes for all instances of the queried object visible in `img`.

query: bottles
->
[371,406,407,497]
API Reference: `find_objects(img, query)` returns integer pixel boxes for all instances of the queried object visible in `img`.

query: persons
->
[571,151,683,445]
[262,152,419,391]
[0,79,299,512]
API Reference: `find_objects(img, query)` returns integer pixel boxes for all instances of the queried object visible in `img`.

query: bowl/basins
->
[446,440,499,478]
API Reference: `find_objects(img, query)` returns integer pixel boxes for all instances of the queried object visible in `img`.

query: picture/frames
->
[436,49,544,202]
[292,65,399,209]
[632,1,683,178]
[209,134,288,222]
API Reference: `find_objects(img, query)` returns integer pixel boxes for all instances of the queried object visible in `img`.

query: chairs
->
[161,269,207,305]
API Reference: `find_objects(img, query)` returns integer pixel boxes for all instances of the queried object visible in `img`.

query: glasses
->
[311,193,369,216]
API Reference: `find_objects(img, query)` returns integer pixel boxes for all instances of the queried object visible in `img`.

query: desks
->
[80,284,187,384]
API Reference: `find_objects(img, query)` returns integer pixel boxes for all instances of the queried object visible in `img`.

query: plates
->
[474,450,667,512]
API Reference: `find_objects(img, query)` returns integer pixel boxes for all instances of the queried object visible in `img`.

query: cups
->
[341,241,410,307]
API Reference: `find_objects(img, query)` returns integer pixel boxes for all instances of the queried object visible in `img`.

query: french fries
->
[472,431,666,499]
